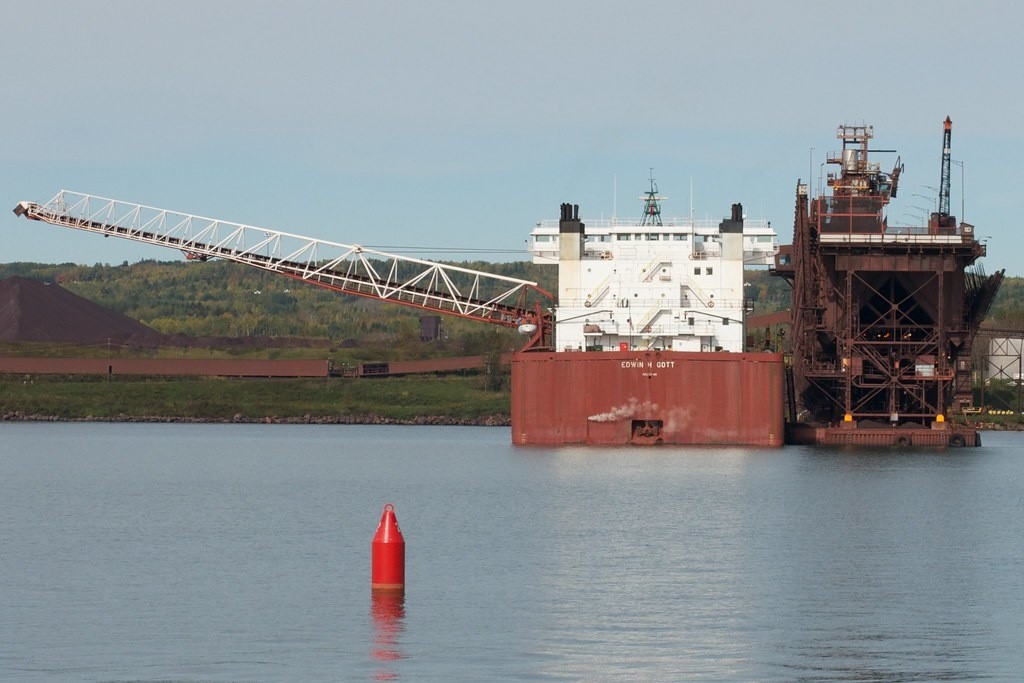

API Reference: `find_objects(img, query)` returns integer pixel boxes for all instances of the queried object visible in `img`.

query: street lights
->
[907,205,930,223]
[937,156,964,227]
[913,193,936,214]
[919,185,938,233]
[902,213,924,230]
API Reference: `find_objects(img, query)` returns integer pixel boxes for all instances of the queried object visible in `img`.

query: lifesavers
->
[584,301,591,308]
[949,434,965,448]
[707,302,714,309]
[896,435,911,449]
